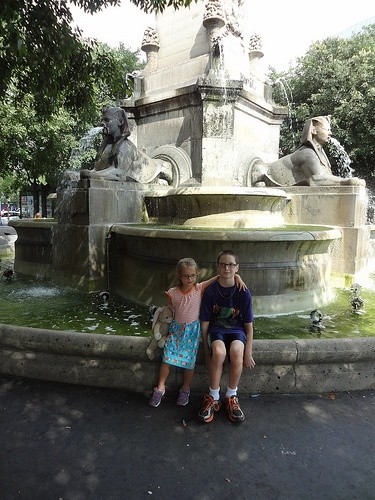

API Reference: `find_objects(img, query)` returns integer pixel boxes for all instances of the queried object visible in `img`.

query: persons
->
[199,249,256,422]
[35,210,41,218]
[152,258,248,407]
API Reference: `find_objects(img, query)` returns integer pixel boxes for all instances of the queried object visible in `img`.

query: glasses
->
[218,262,237,269]
[180,274,198,279]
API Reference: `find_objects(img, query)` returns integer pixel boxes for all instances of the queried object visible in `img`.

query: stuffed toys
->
[145,305,175,360]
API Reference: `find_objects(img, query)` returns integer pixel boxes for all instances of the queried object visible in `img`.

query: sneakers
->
[224,395,245,423]
[198,394,222,424]
[149,385,165,407]
[177,389,190,405]
